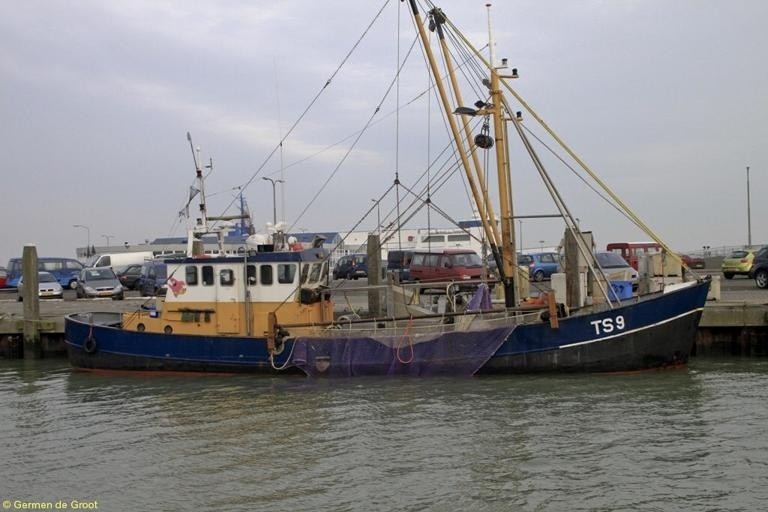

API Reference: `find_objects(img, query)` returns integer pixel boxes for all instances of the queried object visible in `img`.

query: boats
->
[58,0,712,380]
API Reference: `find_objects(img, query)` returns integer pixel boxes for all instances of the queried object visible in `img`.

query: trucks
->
[84,251,154,284]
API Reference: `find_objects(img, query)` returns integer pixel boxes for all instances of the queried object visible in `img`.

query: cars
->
[584,249,641,292]
[75,266,125,300]
[15,270,65,300]
[516,250,565,282]
[720,244,768,290]
[139,260,170,298]
[333,253,368,281]
[118,264,144,288]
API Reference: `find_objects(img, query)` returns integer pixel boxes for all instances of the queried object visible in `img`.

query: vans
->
[386,247,501,294]
[5,255,85,290]
[606,241,690,275]
